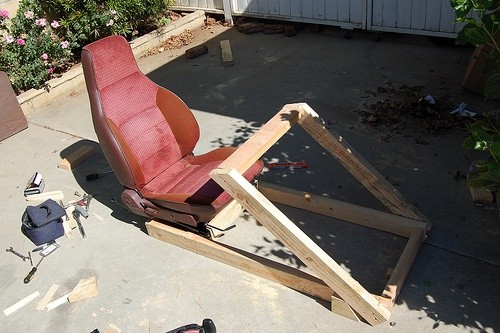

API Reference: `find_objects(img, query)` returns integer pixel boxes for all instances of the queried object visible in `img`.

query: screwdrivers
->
[23,255,45,284]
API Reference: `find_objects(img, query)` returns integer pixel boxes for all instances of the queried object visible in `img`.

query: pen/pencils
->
[32,240,55,252]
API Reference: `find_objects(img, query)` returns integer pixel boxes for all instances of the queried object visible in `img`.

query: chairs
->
[81,36,265,228]
